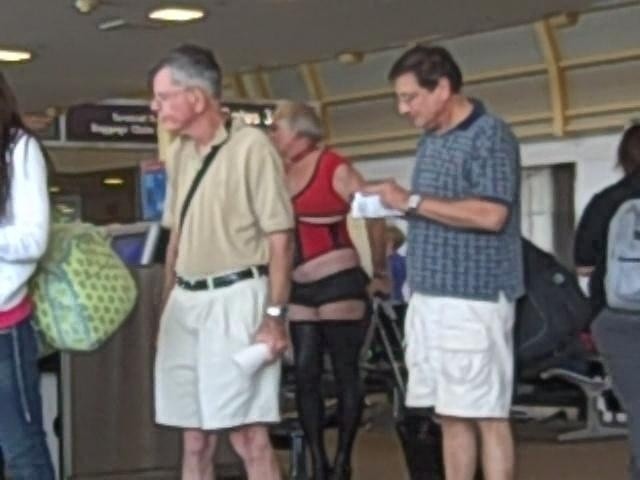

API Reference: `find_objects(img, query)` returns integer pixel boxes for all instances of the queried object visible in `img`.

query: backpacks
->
[604,199,639,312]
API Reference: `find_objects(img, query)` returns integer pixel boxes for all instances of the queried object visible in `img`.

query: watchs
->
[406,192,424,216]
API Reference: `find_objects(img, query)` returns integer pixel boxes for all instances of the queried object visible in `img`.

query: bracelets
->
[265,304,287,319]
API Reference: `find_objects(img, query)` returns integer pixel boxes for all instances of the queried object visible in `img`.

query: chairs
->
[369,302,629,441]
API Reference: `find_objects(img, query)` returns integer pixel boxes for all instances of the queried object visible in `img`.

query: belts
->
[175,266,268,290]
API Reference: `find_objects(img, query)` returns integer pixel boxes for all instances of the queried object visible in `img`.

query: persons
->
[359,42,528,479]
[573,123,639,479]
[376,226,408,362]
[0,73,56,480]
[142,42,297,480]
[268,99,391,479]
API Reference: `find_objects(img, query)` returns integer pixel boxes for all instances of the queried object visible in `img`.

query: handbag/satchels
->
[27,221,139,352]
